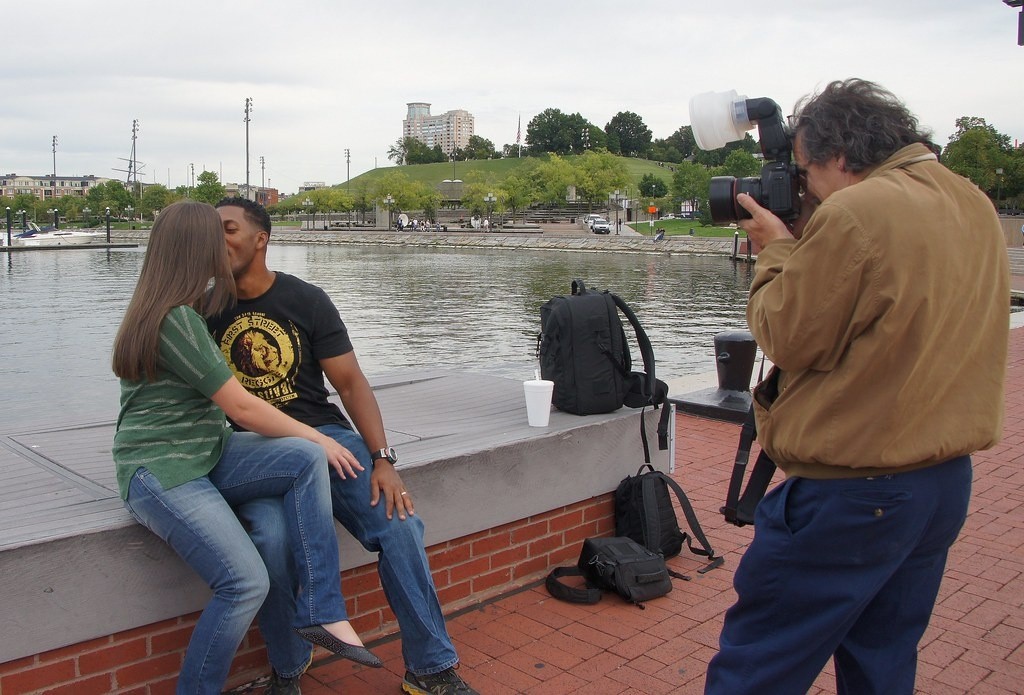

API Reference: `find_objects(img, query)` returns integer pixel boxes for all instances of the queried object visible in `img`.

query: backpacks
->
[614,463,724,580]
[536,279,669,416]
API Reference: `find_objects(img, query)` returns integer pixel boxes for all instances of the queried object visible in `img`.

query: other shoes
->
[295,626,383,668]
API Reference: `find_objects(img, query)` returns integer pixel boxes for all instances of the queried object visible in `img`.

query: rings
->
[401,491,406,496]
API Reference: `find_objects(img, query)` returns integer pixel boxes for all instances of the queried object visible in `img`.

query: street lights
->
[614,189,620,235]
[484,192,497,233]
[131,118,140,182]
[260,156,266,201]
[51,135,58,198]
[244,96,253,199]
[344,149,352,230]
[191,163,194,186]
[383,195,395,230]
[302,198,314,231]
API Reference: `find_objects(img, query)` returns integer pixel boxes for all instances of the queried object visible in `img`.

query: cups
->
[522,379,554,427]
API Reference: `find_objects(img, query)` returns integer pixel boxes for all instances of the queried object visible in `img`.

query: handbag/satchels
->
[578,535,673,610]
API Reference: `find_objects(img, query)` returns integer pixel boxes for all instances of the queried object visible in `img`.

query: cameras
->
[689,89,801,224]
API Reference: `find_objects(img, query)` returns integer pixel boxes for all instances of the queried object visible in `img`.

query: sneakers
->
[263,651,313,695]
[401,662,479,695]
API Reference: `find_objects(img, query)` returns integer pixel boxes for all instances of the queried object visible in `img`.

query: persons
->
[111,199,383,695]
[704,78,1010,695]
[619,219,621,231]
[484,217,488,230]
[396,217,405,232]
[409,218,430,232]
[656,161,677,172]
[434,221,441,232]
[195,198,482,695]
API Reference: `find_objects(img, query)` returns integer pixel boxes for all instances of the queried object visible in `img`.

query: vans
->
[584,214,588,223]
[593,218,610,235]
[587,214,600,229]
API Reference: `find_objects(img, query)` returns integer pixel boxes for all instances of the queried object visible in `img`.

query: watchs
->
[371,446,399,465]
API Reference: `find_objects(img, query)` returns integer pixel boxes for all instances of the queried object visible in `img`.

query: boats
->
[10,230,111,247]
